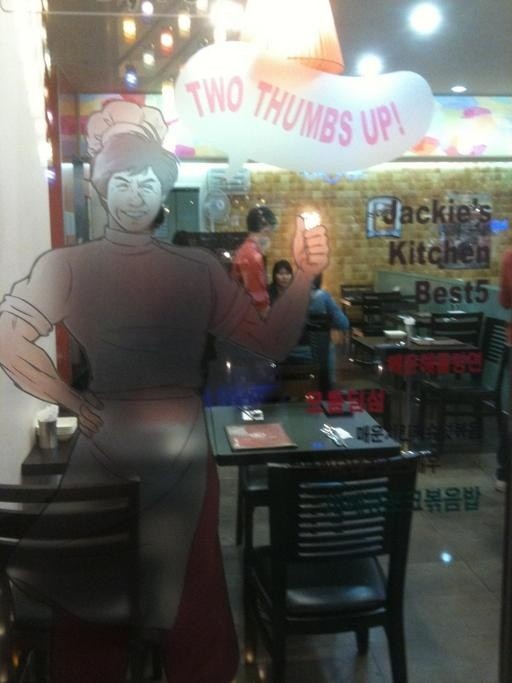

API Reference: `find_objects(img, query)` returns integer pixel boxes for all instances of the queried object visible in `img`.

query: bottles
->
[403,316,417,336]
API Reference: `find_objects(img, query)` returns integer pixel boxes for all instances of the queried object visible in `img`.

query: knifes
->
[322,422,351,448]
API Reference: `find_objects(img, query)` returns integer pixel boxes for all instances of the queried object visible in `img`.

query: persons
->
[0,98,333,681]
[492,240,511,496]
[228,203,280,316]
[269,251,295,300]
[301,271,350,337]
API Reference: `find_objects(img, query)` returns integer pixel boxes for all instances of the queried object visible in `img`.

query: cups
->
[38,419,57,450]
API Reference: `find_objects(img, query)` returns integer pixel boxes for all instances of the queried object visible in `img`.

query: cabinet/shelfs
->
[173,229,267,273]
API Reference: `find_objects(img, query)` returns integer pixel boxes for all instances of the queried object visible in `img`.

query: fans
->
[201,187,234,230]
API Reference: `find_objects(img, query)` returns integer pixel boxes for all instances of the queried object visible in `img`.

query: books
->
[221,421,300,453]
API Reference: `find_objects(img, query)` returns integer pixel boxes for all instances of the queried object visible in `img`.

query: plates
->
[382,329,407,339]
[34,415,78,443]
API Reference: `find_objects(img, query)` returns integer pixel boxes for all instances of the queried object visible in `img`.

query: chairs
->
[237,440,426,682]
[222,340,322,555]
[0,472,166,681]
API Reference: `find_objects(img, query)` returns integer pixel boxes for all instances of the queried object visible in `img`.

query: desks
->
[20,390,410,636]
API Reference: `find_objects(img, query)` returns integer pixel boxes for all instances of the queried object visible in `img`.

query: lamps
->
[123,62,138,84]
